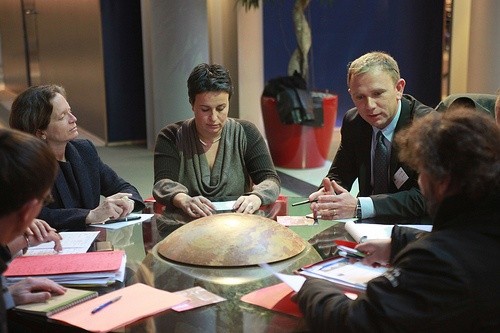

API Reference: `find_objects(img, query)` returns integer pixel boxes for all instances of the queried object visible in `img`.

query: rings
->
[249,203,253,209]
[334,210,336,216]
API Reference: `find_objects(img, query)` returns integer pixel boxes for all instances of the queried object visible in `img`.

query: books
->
[345,220,394,243]
[1,250,127,286]
[15,288,98,317]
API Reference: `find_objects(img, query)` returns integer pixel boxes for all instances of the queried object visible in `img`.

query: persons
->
[0,127,67,333]
[5,219,63,262]
[290,108,500,333]
[153,63,281,218]
[9,84,145,229]
[309,52,436,220]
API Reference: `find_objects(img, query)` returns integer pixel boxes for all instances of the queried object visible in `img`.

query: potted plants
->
[236,0,338,169]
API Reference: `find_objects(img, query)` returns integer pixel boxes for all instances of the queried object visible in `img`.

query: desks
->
[9,197,389,332]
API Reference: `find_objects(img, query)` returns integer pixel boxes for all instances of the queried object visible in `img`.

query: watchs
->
[357,198,362,218]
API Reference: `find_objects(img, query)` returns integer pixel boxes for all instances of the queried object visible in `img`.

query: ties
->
[373,130,389,196]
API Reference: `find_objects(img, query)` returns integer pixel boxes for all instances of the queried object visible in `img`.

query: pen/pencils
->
[103,216,141,224]
[92,295,121,314]
[292,200,319,207]
[337,245,382,267]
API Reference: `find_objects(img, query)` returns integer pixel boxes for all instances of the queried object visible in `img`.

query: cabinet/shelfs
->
[0,0,213,149]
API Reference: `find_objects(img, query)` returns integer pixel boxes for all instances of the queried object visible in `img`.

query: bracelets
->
[24,233,29,247]
